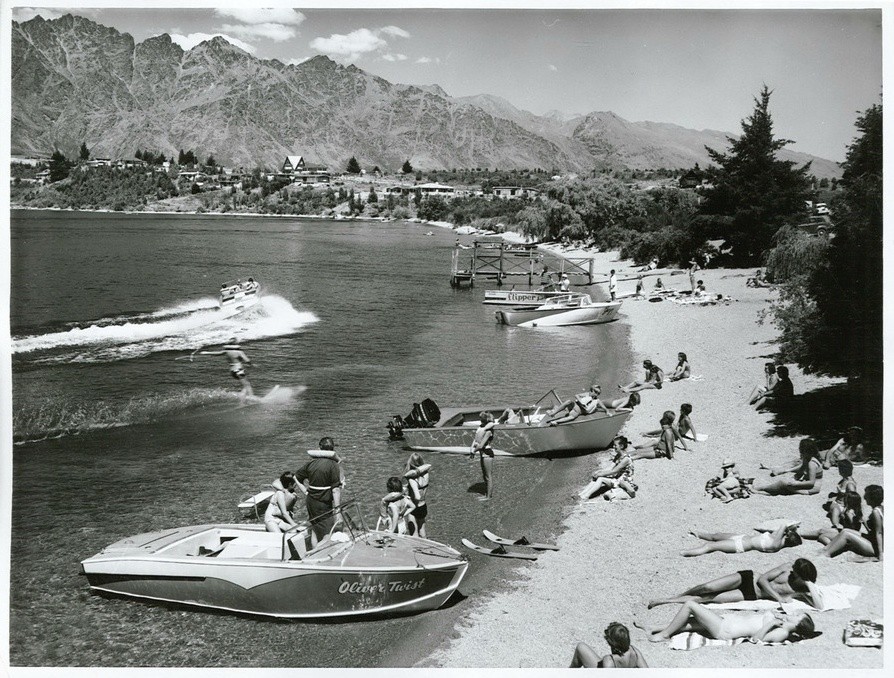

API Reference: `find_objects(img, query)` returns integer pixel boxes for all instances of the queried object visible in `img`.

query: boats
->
[482,280,625,328]
[384,386,633,457]
[218,280,261,306]
[80,497,471,621]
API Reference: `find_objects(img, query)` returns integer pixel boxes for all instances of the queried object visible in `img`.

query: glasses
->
[593,389,600,395]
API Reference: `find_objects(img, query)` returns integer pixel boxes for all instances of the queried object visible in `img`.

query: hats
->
[721,459,735,468]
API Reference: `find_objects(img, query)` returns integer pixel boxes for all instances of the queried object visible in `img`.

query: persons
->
[679,523,802,557]
[633,599,823,643]
[384,452,430,539]
[264,437,344,542]
[568,621,649,668]
[752,439,830,496]
[648,558,817,610]
[713,458,741,504]
[220,278,257,299]
[759,426,866,476]
[196,337,254,407]
[747,363,794,413]
[469,411,495,502]
[549,352,699,502]
[745,270,774,289]
[688,257,705,296]
[829,459,858,530]
[635,257,665,301]
[797,484,884,562]
[541,266,570,298]
[609,269,616,301]
